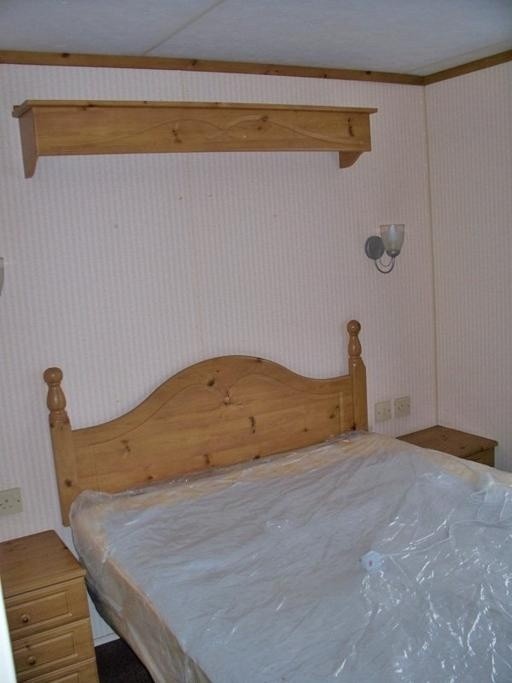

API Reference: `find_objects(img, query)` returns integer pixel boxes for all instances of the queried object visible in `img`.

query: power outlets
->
[0,486,23,515]
[375,399,392,422]
[394,395,411,418]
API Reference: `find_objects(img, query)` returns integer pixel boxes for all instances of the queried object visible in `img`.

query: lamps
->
[365,222,406,275]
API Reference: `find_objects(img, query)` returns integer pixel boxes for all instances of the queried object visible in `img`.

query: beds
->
[41,319,511,681]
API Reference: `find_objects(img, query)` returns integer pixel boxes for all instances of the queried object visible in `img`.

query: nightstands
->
[396,423,499,466]
[1,528,100,681]
